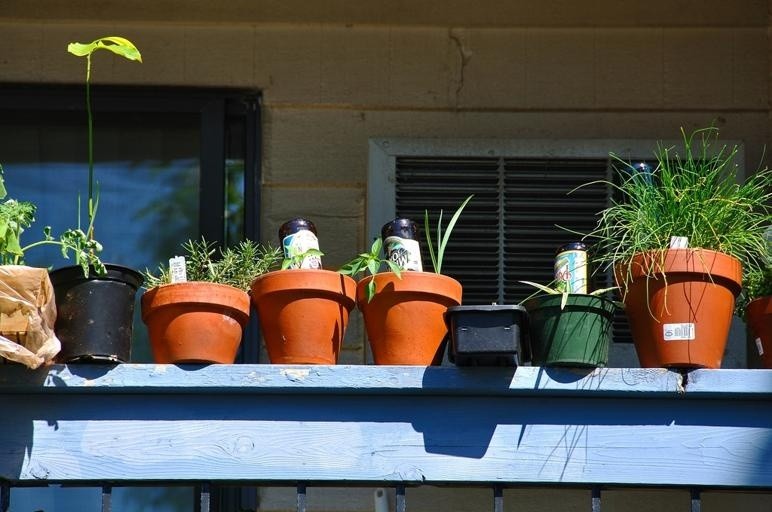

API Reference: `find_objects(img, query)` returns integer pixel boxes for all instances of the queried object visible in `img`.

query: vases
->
[446,276,558,361]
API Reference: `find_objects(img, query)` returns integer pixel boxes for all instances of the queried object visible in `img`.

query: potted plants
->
[140,237,283,364]
[733,225,772,369]
[355,190,476,364]
[566,116,772,368]
[47,35,144,364]
[0,168,107,369]
[518,240,623,369]
[251,217,402,366]
